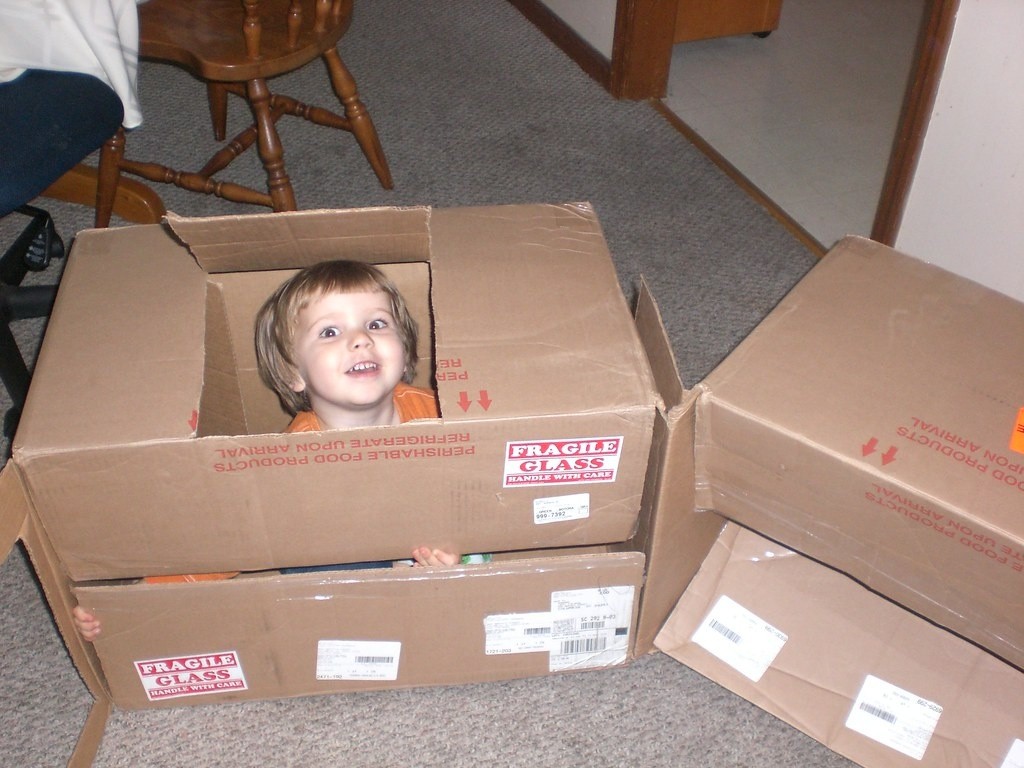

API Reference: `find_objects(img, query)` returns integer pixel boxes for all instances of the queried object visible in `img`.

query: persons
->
[0,1,144,217]
[73,260,491,637]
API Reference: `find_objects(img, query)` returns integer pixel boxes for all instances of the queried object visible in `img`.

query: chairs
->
[1,68,124,445]
[39,0,395,229]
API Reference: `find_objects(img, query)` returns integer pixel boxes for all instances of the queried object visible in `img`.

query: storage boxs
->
[0,202,1024,768]
